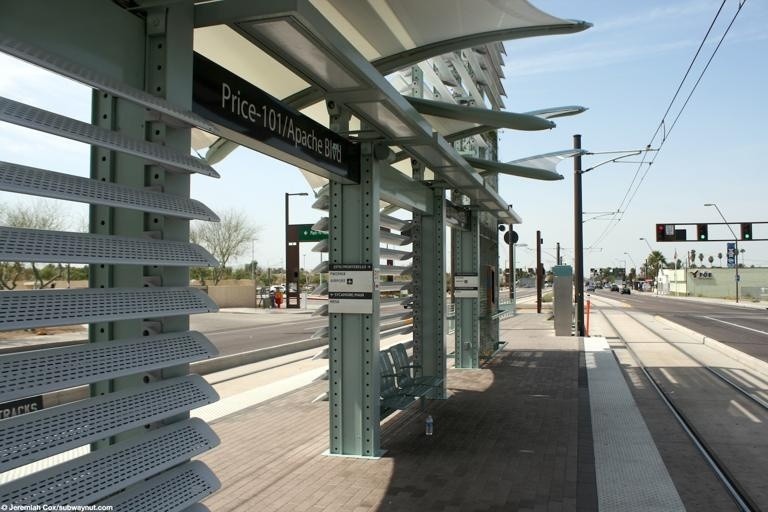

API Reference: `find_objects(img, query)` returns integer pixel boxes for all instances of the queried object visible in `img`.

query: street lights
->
[283,189,310,311]
[704,202,741,304]
[638,237,659,295]
[623,252,636,267]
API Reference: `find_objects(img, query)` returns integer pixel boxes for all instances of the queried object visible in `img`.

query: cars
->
[585,280,631,296]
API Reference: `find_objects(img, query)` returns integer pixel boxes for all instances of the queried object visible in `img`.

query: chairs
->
[380,396,417,410]
[389,343,444,387]
[378,351,433,398]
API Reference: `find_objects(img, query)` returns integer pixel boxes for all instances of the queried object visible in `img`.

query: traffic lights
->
[740,221,752,242]
[656,223,665,243]
[696,223,708,240]
